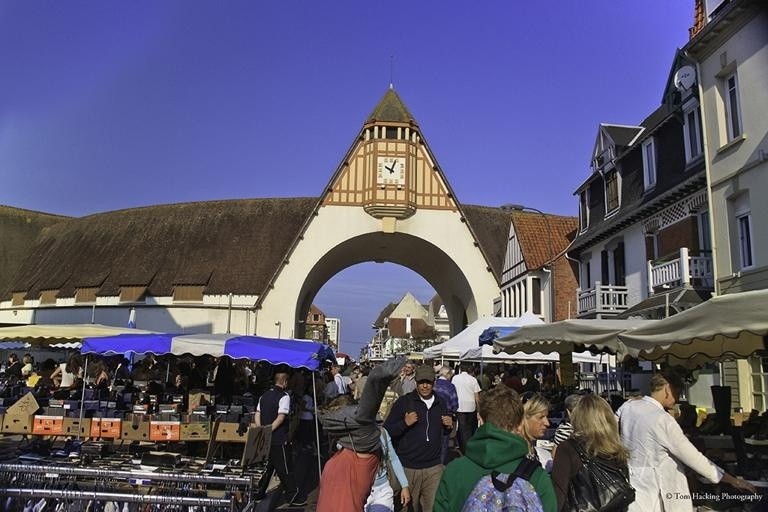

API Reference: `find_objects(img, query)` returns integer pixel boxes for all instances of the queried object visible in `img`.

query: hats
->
[415,365,435,382]
[564,394,579,413]
[660,362,697,388]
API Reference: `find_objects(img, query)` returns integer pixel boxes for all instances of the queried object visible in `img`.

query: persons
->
[613,369,757,512]
[0,351,630,512]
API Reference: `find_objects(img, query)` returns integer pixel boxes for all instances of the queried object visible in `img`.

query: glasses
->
[522,390,536,404]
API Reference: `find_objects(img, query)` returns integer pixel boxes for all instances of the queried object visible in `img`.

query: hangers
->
[0,463,249,511]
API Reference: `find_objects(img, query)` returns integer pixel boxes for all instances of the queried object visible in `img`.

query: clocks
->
[377,156,405,185]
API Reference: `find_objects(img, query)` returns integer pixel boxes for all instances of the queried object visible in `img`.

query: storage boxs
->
[0,399,250,443]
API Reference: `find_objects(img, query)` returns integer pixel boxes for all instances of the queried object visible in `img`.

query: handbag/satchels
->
[561,459,635,512]
[460,458,544,512]
[386,459,402,496]
